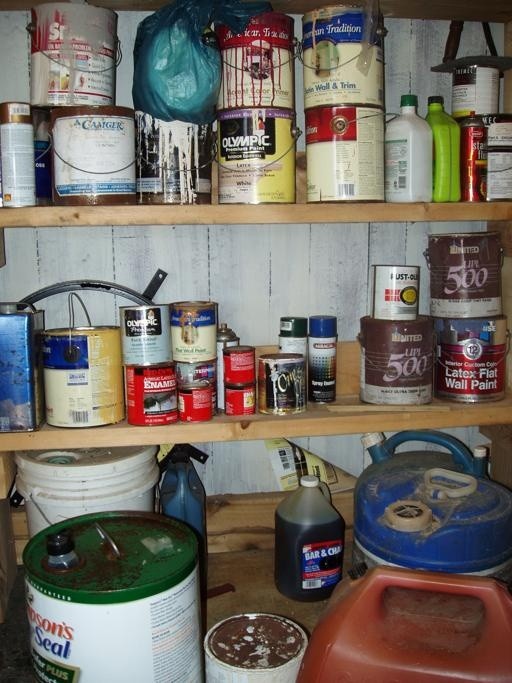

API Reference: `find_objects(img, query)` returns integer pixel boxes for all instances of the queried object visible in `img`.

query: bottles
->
[386,94,433,203]
[424,94,461,202]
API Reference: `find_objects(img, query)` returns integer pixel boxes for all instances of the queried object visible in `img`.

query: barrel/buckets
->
[214,13,298,110]
[305,105,397,202]
[298,4,387,108]
[50,105,134,206]
[13,447,160,535]
[159,448,207,530]
[358,315,436,404]
[483,112,512,201]
[214,109,301,203]
[424,233,504,317]
[40,292,125,429]
[274,475,346,602]
[352,429,512,578]
[25,3,122,107]
[20,511,205,681]
[433,318,507,405]
[135,110,214,206]
[295,565,512,681]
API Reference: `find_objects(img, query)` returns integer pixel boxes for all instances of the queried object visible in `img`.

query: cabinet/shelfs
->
[1,1,512,677]
